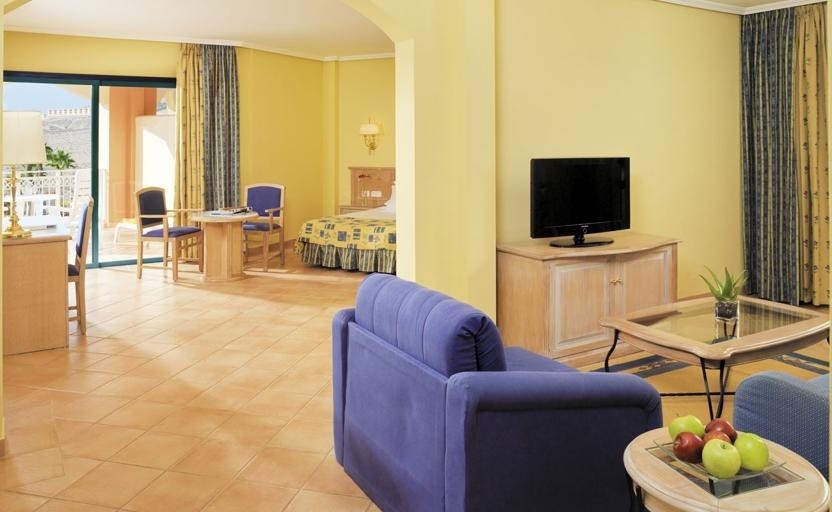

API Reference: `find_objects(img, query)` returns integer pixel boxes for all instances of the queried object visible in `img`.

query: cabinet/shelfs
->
[496,227,685,368]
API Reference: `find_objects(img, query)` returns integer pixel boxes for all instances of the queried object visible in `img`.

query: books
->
[211,206,252,216]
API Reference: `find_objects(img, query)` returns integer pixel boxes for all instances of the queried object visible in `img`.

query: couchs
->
[329,267,664,512]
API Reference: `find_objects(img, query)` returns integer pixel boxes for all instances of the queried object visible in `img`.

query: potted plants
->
[696,263,752,324]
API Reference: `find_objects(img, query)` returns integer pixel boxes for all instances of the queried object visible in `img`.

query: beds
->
[293,165,396,275]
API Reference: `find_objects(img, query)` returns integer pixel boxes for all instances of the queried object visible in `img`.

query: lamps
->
[3,109,49,239]
[357,116,385,152]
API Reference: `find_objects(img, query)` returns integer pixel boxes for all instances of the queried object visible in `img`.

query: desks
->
[190,208,259,284]
[621,424,829,511]
[2,202,73,355]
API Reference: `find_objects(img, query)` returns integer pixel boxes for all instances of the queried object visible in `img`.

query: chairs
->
[242,182,286,272]
[731,371,830,482]
[68,193,95,335]
[133,186,204,282]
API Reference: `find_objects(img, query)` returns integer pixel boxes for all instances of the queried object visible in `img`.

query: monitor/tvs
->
[530,157,629,247]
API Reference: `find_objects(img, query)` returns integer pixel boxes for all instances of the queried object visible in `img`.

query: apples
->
[705,418,737,443]
[673,432,704,463]
[734,432,768,471]
[669,415,705,440]
[703,430,732,444]
[702,439,741,479]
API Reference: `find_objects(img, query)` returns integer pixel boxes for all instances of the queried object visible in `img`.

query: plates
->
[654,438,787,483]
